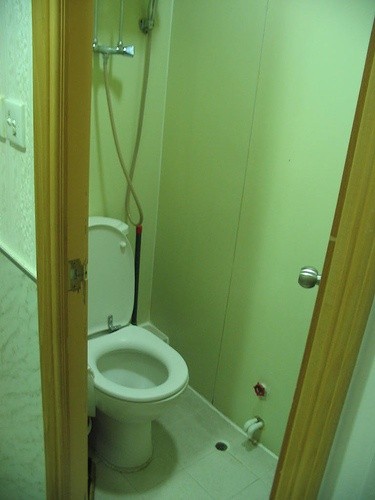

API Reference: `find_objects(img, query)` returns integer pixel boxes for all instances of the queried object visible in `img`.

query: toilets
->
[87,215,190,474]
